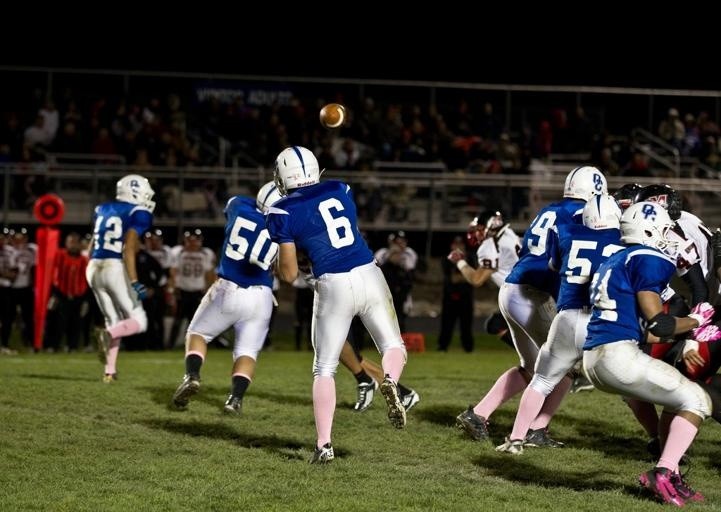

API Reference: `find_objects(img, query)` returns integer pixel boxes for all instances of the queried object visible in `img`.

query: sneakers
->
[524,424,565,447]
[639,466,704,506]
[354,376,379,411]
[224,394,242,413]
[92,326,112,364]
[380,374,420,429]
[174,372,201,407]
[310,442,334,463]
[496,436,524,454]
[457,404,490,440]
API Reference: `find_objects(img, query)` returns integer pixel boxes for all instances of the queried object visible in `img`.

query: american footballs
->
[320,103,345,129]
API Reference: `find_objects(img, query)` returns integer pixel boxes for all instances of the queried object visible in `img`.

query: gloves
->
[688,301,721,343]
[447,248,464,263]
[132,281,147,300]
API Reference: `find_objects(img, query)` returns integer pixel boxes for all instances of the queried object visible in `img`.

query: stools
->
[400,333,425,353]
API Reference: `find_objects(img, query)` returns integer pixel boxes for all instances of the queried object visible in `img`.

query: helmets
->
[468,208,505,249]
[256,146,320,211]
[563,166,681,259]
[115,174,156,213]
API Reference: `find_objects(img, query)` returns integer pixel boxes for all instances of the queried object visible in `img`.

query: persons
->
[339,246,421,414]
[173,179,281,417]
[449,165,720,508]
[84,174,157,382]
[2,93,721,223]
[0,229,105,354]
[266,146,408,464]
[127,231,231,350]
[293,228,475,352]
